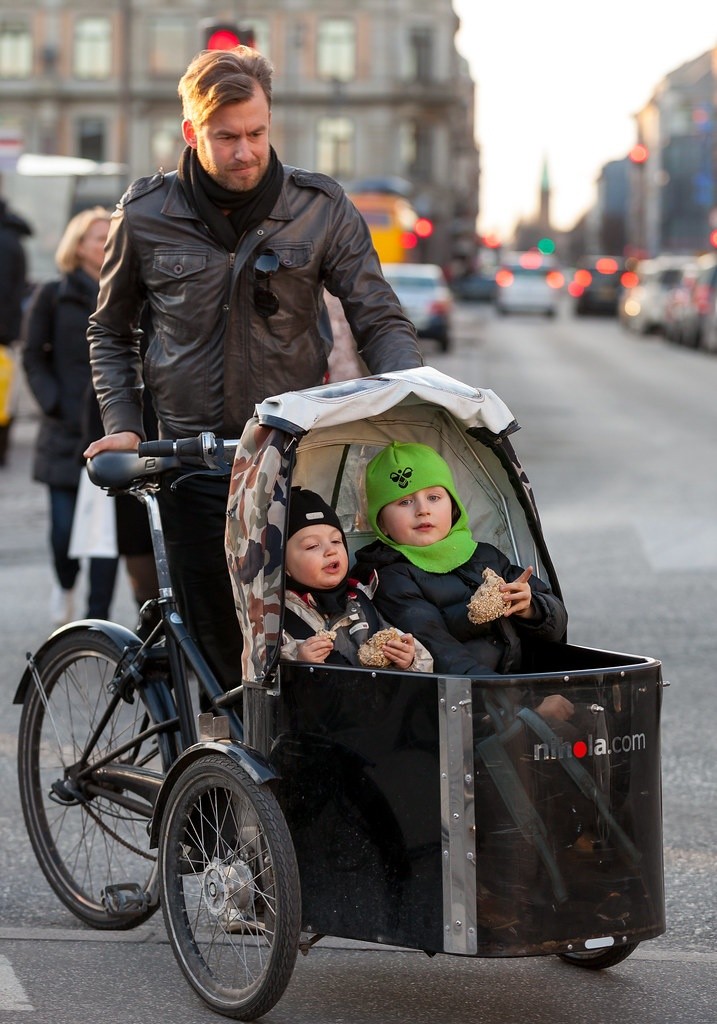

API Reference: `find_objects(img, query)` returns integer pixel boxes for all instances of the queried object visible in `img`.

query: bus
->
[347,195,421,263]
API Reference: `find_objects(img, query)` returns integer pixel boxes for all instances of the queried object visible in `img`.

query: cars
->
[379,262,453,354]
[499,254,557,317]
[574,252,717,352]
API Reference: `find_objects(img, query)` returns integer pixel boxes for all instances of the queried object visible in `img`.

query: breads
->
[466,568,511,625]
[315,628,336,642]
[357,627,402,670]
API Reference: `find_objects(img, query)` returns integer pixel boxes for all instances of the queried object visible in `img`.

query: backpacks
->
[472,689,619,912]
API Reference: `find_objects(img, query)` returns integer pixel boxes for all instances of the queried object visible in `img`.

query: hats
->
[283,485,346,546]
[365,442,477,574]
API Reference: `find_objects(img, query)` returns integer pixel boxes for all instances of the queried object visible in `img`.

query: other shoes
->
[49,584,73,626]
[220,846,267,936]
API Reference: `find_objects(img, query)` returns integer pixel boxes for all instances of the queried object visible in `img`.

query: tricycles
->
[13,431,667,1021]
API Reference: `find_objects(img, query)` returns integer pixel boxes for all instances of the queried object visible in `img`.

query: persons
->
[0,193,157,621]
[349,441,630,944]
[280,486,433,673]
[85,45,425,931]
